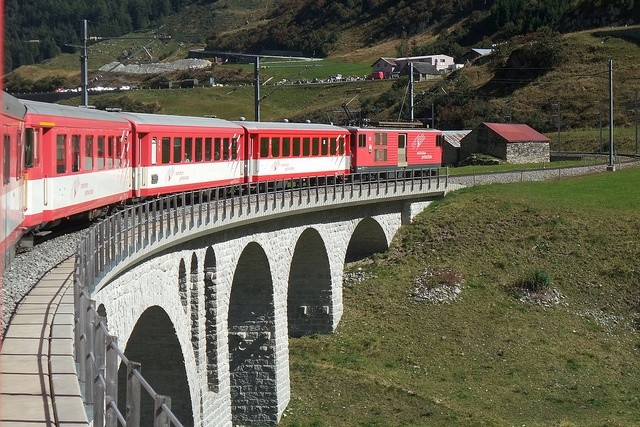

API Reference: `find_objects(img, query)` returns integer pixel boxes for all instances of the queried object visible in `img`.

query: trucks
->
[181,79,199,88]
[157,81,172,89]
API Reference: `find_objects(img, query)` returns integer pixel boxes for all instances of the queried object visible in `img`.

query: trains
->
[0,89,442,275]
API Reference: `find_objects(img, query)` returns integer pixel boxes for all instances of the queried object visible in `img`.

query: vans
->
[368,71,384,79]
